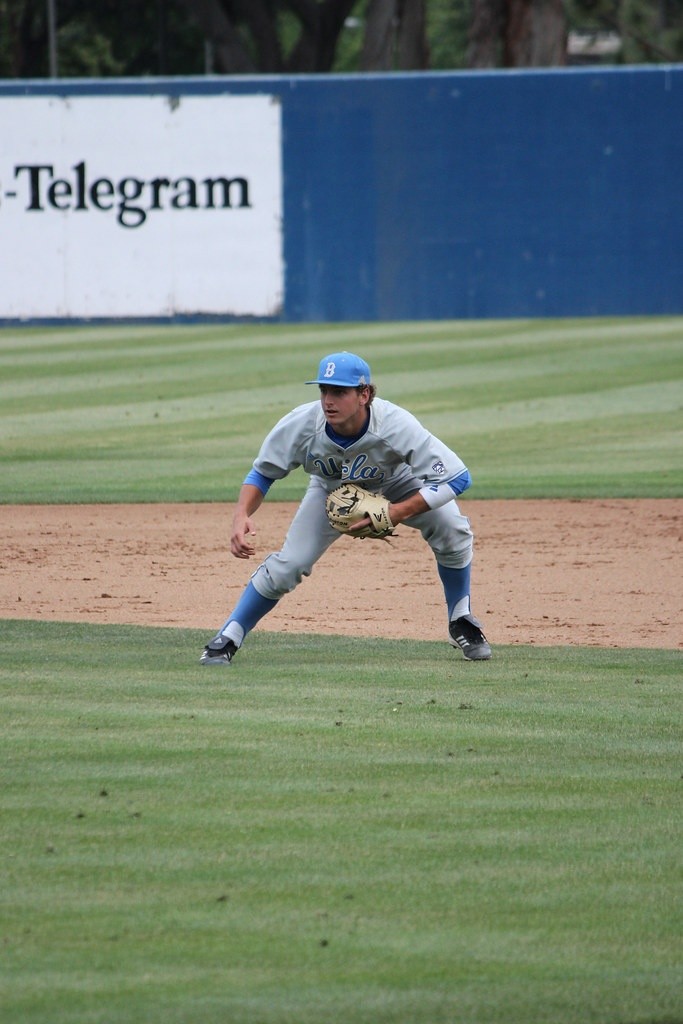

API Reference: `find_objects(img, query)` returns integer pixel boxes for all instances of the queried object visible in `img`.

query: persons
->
[197,348,493,667]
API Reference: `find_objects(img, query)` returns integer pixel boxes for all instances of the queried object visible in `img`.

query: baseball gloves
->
[325,482,399,540]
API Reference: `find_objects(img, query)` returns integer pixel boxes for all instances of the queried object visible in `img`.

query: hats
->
[305,351,370,387]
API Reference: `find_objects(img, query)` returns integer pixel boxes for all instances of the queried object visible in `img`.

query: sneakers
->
[448,613,492,660]
[199,634,238,666]
